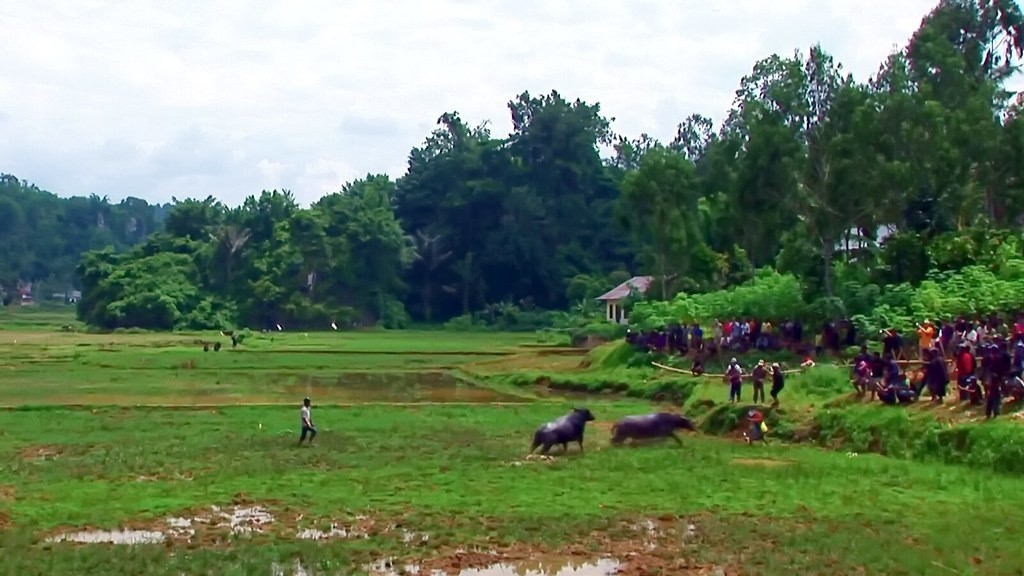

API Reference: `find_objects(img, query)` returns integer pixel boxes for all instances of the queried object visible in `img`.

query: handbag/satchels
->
[760,421,768,433]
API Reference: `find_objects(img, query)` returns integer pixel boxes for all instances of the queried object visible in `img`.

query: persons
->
[725,357,784,446]
[298,397,317,447]
[705,316,856,358]
[625,323,705,377]
[800,355,815,368]
[850,311,1024,419]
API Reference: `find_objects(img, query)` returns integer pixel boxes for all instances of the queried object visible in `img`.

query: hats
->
[772,362,779,367]
[858,361,866,369]
[729,357,737,362]
[758,360,764,365]
[988,344,998,349]
[966,376,977,382]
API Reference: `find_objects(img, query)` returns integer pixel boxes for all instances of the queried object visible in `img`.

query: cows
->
[528,406,595,455]
[610,414,697,447]
